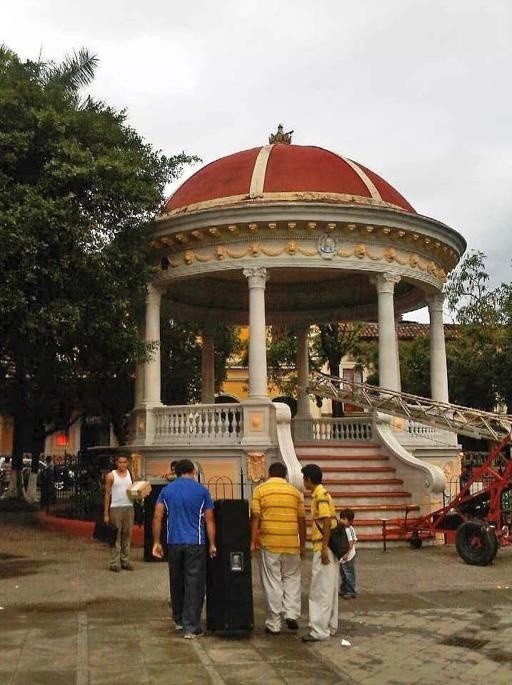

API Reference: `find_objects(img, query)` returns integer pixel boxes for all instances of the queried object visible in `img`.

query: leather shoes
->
[122,563,133,570]
[111,565,119,572]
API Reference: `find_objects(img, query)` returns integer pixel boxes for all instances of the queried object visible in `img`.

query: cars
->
[0,455,77,491]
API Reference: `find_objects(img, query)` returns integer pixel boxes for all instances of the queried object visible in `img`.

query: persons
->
[460,466,471,495]
[165,460,178,481]
[338,508,357,599]
[104,454,150,572]
[0,453,105,512]
[249,462,306,634]
[150,459,217,638]
[300,464,350,642]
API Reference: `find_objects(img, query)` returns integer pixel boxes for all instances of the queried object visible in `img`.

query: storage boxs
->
[127,479,151,500]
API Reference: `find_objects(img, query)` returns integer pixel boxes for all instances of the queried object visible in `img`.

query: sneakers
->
[184,627,205,639]
[265,627,280,634]
[338,591,355,599]
[286,618,299,629]
[302,634,319,641]
[174,623,184,630]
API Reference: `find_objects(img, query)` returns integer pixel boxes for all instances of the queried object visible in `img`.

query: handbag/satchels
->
[325,520,351,560]
[94,520,118,545]
